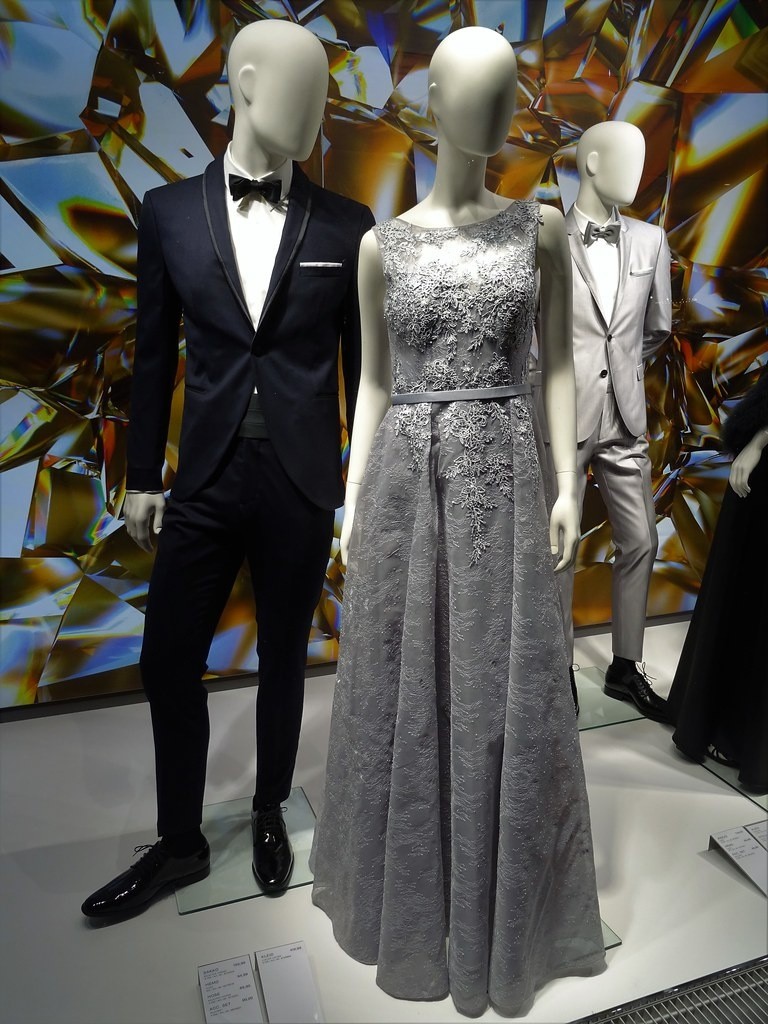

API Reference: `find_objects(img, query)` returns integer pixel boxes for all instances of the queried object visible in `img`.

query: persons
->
[531,121,670,726]
[79,19,376,920]
[307,27,607,1023]
[663,361,768,796]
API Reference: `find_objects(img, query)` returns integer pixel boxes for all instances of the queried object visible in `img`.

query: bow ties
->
[229,173,283,205]
[583,221,621,248]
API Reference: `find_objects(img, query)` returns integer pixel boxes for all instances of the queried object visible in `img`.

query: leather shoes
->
[250,805,295,893]
[604,661,678,726]
[80,833,211,918]
[569,663,581,718]
